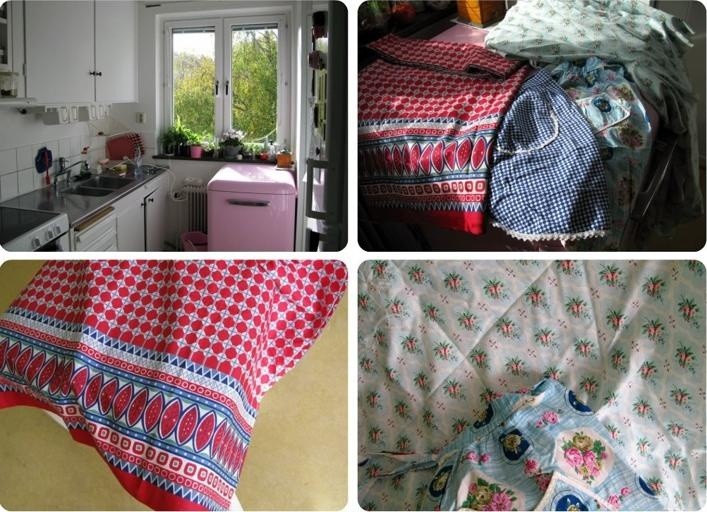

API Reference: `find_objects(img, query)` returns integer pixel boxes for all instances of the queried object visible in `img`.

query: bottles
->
[1,71,19,98]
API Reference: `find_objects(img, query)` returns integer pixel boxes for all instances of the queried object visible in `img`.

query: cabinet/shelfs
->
[111,171,174,252]
[24,1,140,126]
[0,0,25,112]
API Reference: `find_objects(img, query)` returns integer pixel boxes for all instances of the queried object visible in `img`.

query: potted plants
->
[275,146,292,168]
[158,126,269,160]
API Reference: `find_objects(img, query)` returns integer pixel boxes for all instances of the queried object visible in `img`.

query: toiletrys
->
[77,144,91,176]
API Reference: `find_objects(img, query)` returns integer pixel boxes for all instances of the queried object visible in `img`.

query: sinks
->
[58,185,115,198]
[77,174,137,190]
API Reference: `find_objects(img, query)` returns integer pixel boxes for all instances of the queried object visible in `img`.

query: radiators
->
[183,184,208,233]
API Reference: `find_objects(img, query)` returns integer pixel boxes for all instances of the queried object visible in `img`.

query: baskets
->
[180,231,208,251]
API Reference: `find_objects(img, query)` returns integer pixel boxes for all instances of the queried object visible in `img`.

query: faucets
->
[53,158,89,185]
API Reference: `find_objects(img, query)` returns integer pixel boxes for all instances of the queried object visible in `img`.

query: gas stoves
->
[0,205,70,251]
